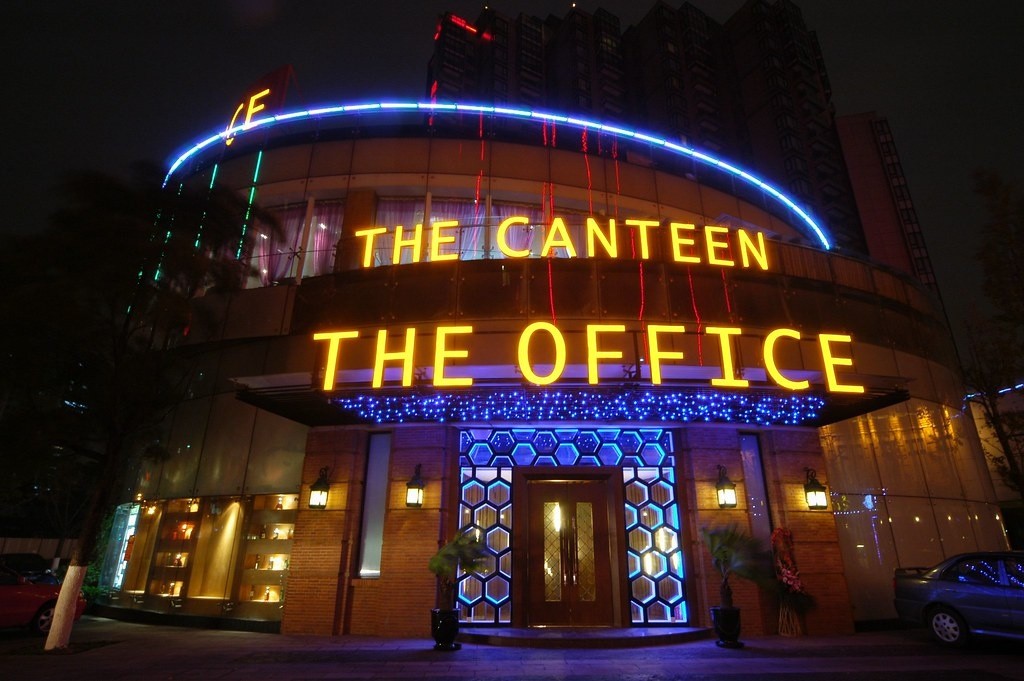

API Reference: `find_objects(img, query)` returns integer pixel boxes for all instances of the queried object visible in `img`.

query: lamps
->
[309,467,329,510]
[405,463,425,509]
[716,463,737,509]
[802,466,829,510]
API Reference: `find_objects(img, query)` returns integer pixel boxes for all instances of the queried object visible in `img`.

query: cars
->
[0,551,88,634]
[895,549,1024,647]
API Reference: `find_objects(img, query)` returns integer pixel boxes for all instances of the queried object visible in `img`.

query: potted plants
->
[425,523,491,652]
[700,519,767,650]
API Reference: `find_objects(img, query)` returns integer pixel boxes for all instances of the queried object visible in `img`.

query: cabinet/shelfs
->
[234,494,299,604]
[143,495,202,596]
